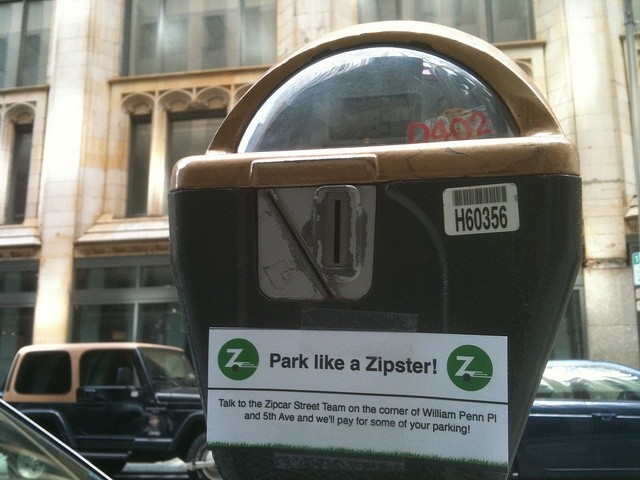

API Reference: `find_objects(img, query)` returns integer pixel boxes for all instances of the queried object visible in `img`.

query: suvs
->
[0,339,227,480]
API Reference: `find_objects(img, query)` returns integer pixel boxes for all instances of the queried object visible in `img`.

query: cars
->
[514,358,640,479]
